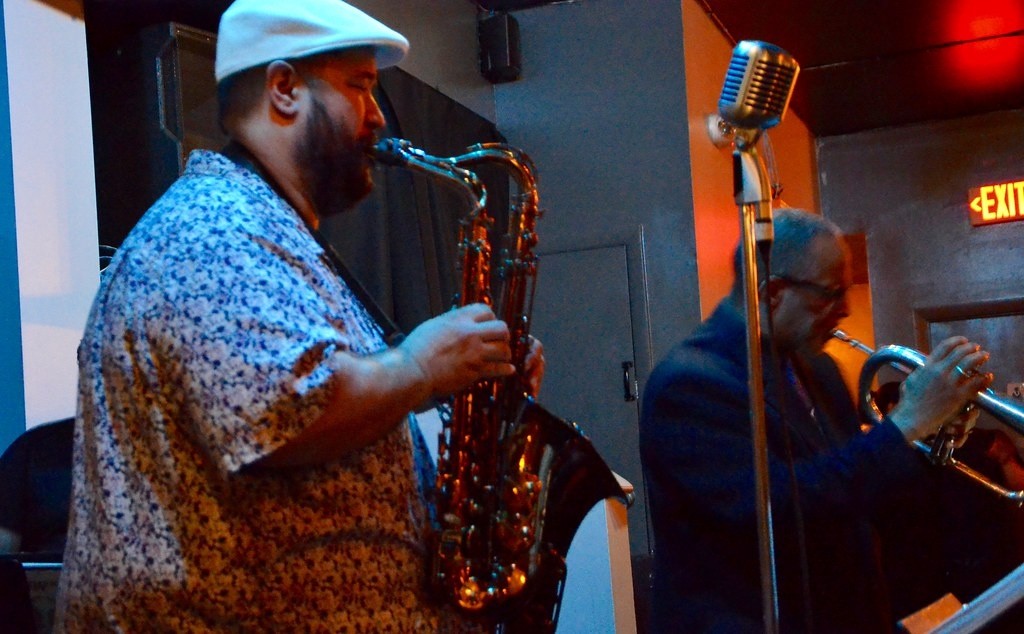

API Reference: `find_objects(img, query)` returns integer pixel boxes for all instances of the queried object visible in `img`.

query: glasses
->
[779,273,848,305]
[959,403,975,415]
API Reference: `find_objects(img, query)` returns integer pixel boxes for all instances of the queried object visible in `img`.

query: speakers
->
[479,14,522,83]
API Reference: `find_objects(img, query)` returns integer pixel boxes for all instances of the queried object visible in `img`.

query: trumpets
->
[833,328,1023,507]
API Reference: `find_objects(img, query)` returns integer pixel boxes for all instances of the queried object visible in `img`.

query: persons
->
[0,417,74,634]
[638,209,995,634]
[875,379,1024,574]
[54,0,548,634]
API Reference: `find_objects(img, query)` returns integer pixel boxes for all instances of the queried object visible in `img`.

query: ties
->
[780,353,823,440]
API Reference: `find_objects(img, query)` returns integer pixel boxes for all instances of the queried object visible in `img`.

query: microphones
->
[717,41,801,223]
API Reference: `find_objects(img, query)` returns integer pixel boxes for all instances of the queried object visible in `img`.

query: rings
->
[955,365,969,378]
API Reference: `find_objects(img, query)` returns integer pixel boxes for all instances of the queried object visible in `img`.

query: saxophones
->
[372,133,632,611]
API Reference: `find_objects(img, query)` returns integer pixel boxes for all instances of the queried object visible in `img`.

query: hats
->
[215,0,409,83]
